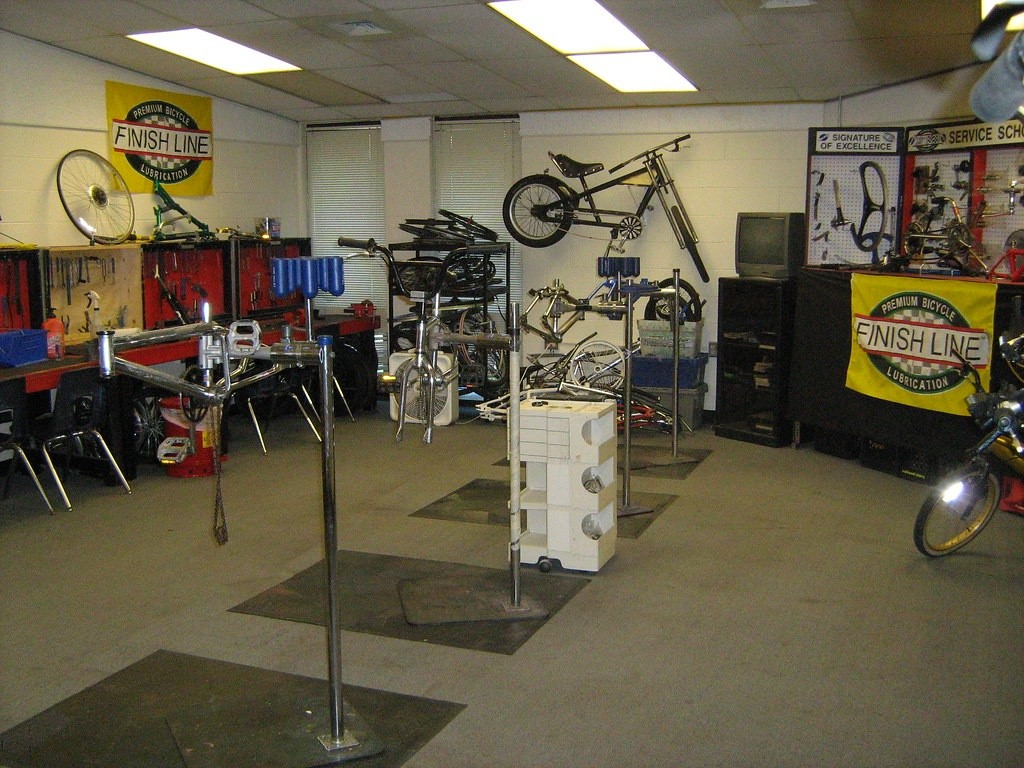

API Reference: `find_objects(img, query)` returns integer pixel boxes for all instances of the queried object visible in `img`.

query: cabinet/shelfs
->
[387,240,511,413]
[319,329,379,417]
[713,275,797,449]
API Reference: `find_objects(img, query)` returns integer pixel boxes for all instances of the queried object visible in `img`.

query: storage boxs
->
[627,352,708,389]
[635,383,709,430]
[637,318,706,359]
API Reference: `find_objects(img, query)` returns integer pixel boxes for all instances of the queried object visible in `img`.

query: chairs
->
[0,367,132,514]
[222,363,323,457]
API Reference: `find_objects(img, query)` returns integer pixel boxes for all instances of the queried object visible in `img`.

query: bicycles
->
[914,348,1023,560]
[502,133,712,284]
